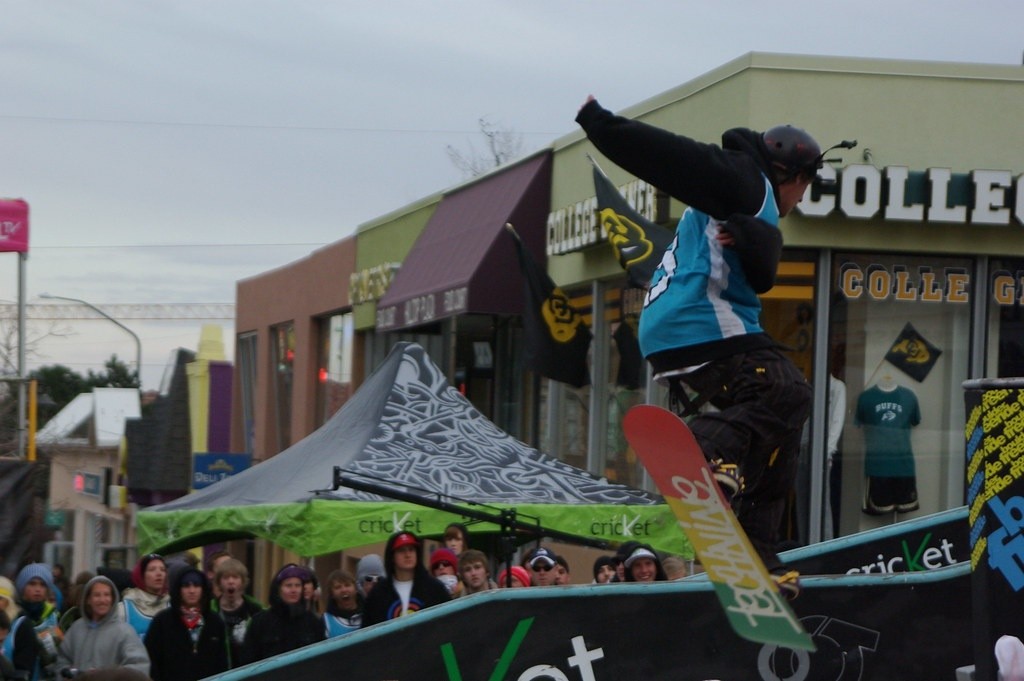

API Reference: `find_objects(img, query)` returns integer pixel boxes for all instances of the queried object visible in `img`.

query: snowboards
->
[622,405,818,654]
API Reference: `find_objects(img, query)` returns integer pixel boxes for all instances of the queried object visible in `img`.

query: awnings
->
[374,150,552,332]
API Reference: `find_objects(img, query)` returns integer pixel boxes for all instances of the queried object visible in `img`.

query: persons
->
[0,521,687,681]
[854,374,921,516]
[575,94,823,599]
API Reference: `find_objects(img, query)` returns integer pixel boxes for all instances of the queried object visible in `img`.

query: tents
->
[135,343,695,579]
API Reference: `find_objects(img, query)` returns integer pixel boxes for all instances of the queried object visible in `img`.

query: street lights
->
[37,293,145,391]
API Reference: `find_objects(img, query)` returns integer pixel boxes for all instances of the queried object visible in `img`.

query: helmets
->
[763,123,824,170]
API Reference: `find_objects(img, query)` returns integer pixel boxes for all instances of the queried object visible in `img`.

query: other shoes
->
[708,459,744,501]
[771,571,800,602]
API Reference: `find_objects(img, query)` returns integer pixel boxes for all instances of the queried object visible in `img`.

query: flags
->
[885,322,942,383]
[591,164,676,287]
[518,241,591,388]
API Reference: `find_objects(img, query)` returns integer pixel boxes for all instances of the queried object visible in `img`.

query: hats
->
[393,534,417,549]
[555,556,569,572]
[428,548,459,576]
[624,549,655,568]
[138,552,168,581]
[498,565,531,587]
[610,540,640,568]
[526,547,555,566]
[593,556,614,583]
[15,561,53,598]
[181,570,201,586]
[443,522,468,547]
[276,565,305,586]
[354,553,387,595]
[0,576,16,607]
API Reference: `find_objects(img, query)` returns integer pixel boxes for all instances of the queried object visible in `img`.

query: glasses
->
[364,574,386,584]
[612,557,630,568]
[431,560,452,570]
[530,563,553,572]
